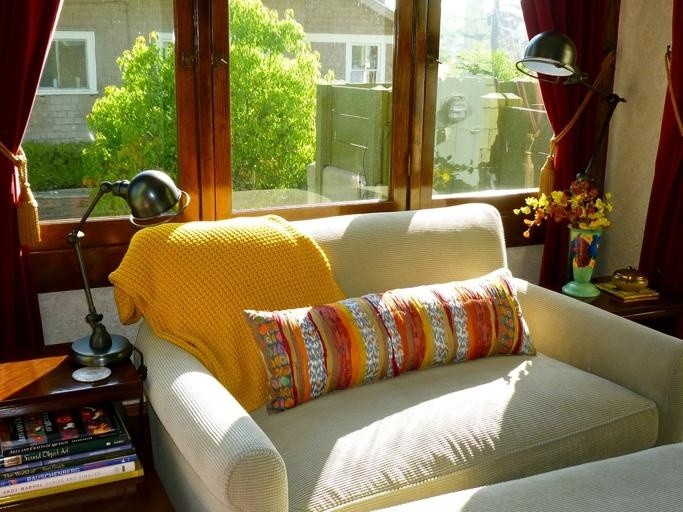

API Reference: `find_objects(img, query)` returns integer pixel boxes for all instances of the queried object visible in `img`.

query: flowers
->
[513,178,614,239]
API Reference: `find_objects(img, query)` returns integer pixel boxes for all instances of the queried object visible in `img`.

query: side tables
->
[0,341,181,511]
[556,277,672,335]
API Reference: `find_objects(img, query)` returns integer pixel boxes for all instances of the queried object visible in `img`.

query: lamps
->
[66,169,191,367]
[517,31,627,183]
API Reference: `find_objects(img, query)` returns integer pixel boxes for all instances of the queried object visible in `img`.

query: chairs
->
[136,203,683,512]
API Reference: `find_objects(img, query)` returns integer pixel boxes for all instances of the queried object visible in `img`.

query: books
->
[0,400,145,504]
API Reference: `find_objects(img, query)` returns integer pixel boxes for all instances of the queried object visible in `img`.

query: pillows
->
[241,265,537,416]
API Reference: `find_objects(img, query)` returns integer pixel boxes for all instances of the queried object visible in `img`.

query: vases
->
[561,225,604,298]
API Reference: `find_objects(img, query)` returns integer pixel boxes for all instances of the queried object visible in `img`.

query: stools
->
[375,441,683,512]
